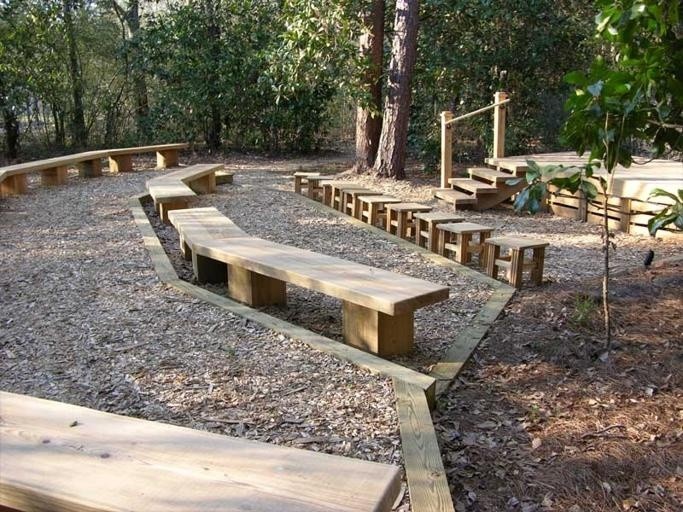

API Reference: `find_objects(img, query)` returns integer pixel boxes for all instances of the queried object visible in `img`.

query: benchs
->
[146,164,224,224]
[0,142,189,200]
[168,206,449,353]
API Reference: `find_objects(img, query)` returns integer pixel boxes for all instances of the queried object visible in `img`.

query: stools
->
[0,389,401,512]
[295,171,549,289]
